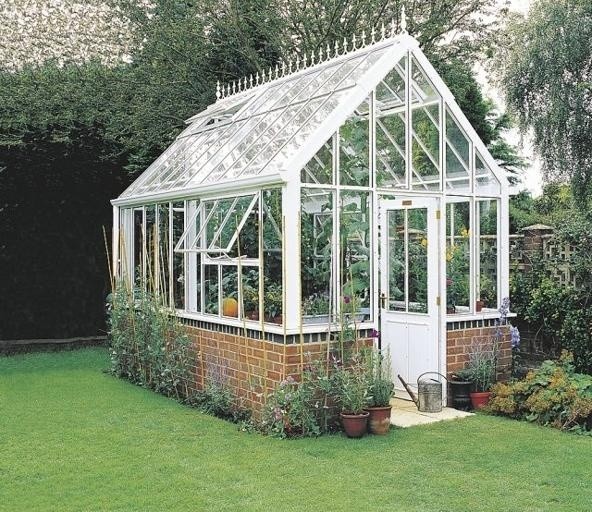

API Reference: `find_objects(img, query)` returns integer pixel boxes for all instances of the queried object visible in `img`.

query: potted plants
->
[454,337,506,412]
[319,342,396,440]
[244,281,282,325]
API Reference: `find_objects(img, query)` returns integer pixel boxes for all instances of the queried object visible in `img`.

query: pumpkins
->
[223,298,238,316]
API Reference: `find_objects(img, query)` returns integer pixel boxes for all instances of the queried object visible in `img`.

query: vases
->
[302,312,365,325]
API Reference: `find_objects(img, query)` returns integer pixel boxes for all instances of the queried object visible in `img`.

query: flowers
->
[300,290,362,315]
